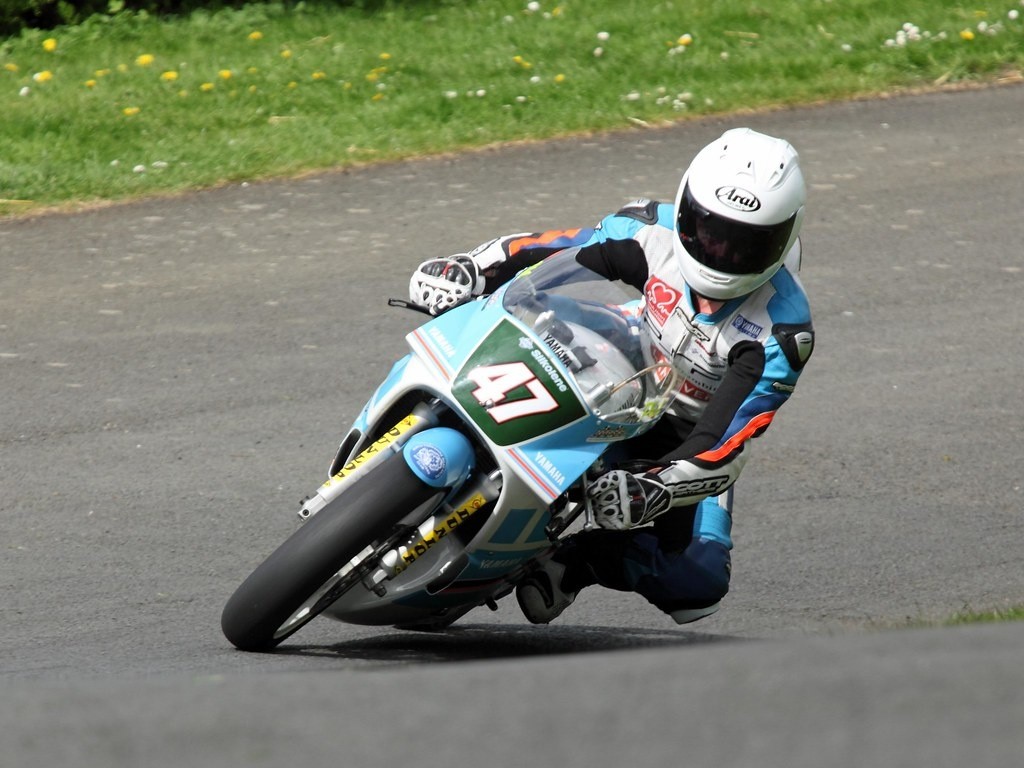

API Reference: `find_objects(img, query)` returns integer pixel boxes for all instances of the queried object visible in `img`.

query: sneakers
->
[516,559,576,624]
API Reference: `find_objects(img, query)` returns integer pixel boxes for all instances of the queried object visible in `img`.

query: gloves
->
[408,255,486,317]
[587,470,672,531]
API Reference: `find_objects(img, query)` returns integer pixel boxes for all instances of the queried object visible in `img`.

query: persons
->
[406,126,817,625]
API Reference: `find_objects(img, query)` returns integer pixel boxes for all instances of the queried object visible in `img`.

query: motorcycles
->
[222,246,694,656]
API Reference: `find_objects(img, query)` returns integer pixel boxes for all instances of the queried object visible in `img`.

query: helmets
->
[674,126,805,300]
